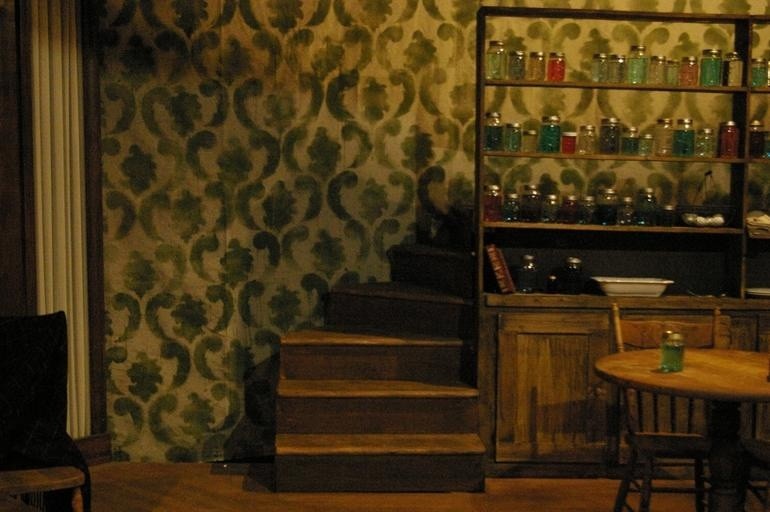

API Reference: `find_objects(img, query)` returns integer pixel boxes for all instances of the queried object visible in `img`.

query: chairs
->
[0,309,92,511]
[608,300,735,511]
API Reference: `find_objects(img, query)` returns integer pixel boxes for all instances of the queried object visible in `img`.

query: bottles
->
[518,251,585,294]
[486,103,768,165]
[658,329,686,373]
[491,37,767,91]
[487,176,770,227]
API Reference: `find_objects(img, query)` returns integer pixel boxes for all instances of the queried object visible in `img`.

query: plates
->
[748,285,768,296]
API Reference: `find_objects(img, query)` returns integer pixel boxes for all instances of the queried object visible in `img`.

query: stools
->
[735,436,770,512]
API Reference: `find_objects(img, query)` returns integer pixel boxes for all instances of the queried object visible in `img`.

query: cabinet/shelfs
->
[475,6,770,483]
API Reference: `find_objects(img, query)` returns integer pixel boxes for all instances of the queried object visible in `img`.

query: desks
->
[592,345,770,511]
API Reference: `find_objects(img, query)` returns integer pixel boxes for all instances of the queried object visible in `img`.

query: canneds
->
[661,331,685,372]
[591,44,743,87]
[749,118,766,157]
[565,257,582,294]
[750,57,767,85]
[508,50,564,80]
[485,184,675,227]
[484,111,739,159]
[517,254,537,293]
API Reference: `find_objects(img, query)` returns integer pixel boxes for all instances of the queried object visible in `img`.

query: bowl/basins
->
[590,270,674,301]
